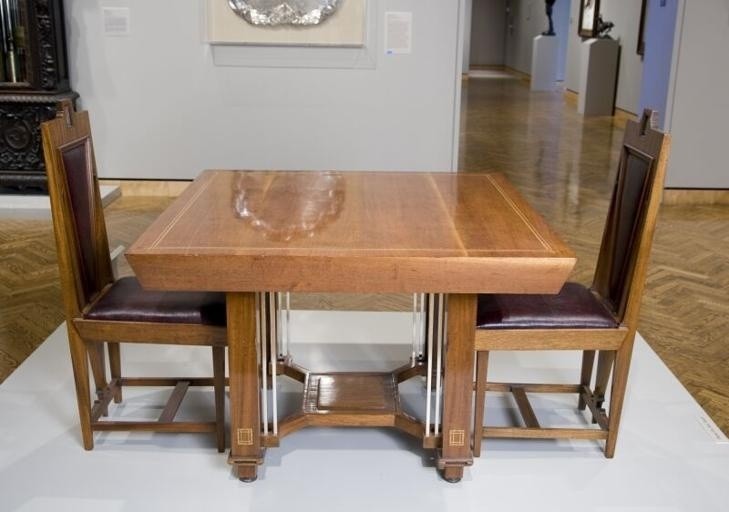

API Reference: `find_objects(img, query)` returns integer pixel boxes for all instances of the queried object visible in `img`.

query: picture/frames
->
[577,0,600,38]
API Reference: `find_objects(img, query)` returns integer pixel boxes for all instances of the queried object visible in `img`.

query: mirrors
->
[0,0,31,82]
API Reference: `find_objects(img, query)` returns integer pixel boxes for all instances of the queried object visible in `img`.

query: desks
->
[125,169,577,483]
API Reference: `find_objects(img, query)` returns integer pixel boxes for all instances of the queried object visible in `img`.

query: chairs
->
[41,98,275,451]
[473,107,671,457]
